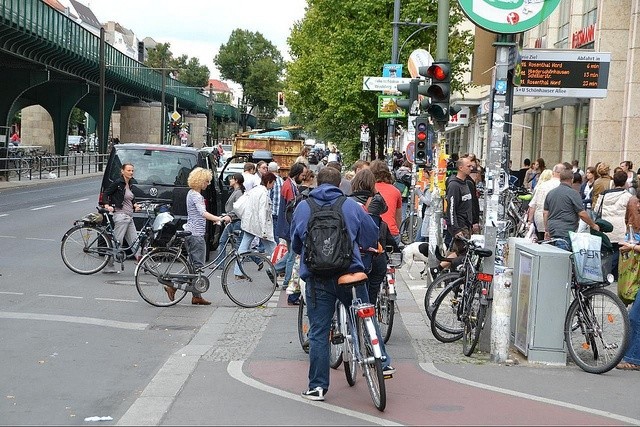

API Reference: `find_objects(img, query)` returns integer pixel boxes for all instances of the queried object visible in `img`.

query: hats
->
[268,162,279,172]
[232,173,244,184]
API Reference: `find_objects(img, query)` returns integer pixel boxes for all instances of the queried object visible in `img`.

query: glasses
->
[260,166,268,169]
[205,181,211,186]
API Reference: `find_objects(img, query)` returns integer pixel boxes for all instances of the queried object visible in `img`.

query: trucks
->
[232,123,307,181]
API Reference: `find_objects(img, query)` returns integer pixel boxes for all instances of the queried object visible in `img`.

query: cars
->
[214,144,232,162]
[68,135,86,153]
[215,167,244,181]
[221,161,257,171]
[307,148,330,165]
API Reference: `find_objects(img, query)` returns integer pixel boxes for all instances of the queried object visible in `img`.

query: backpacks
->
[302,194,353,277]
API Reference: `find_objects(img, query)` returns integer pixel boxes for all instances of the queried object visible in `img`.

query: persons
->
[314,157,328,175]
[445,153,485,183]
[591,162,613,210]
[163,167,232,305]
[535,158,547,185]
[370,160,402,245]
[579,167,600,210]
[102,164,162,274]
[325,148,330,155]
[571,160,584,177]
[508,160,519,187]
[11,130,20,146]
[571,173,583,191]
[519,159,531,186]
[209,173,263,271]
[393,147,412,183]
[266,162,308,289]
[328,161,353,196]
[533,169,553,194]
[254,160,268,187]
[543,170,600,252]
[625,168,640,243]
[267,162,284,238]
[202,143,208,148]
[414,167,432,275]
[187,143,193,147]
[332,146,336,153]
[620,161,638,196]
[616,242,640,370]
[428,158,474,293]
[291,166,396,401]
[295,147,311,169]
[234,173,286,279]
[285,252,306,305]
[352,159,370,175]
[464,175,479,235]
[115,135,120,144]
[241,162,256,194]
[218,143,225,166]
[297,170,314,199]
[523,161,539,191]
[594,171,640,282]
[211,147,220,167]
[528,163,566,240]
[344,169,402,306]
[611,166,629,192]
[319,149,324,156]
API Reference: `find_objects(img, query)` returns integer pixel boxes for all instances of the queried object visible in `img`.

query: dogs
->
[392,242,448,280]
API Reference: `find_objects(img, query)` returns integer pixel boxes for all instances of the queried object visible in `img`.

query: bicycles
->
[386,245,402,281]
[423,234,494,360]
[60,200,190,278]
[337,272,393,412]
[374,267,396,345]
[6,148,58,174]
[536,226,630,373]
[298,294,344,369]
[399,213,418,245]
[134,213,277,308]
[504,185,532,239]
[424,255,505,335]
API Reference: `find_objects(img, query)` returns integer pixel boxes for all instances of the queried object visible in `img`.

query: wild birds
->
[404,16,409,25]
[416,15,422,26]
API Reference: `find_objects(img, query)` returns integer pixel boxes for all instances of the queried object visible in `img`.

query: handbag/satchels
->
[617,245,640,303]
[568,226,604,285]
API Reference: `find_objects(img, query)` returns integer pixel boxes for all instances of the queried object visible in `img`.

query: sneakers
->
[382,362,397,376]
[104,266,120,274]
[300,385,325,401]
[145,262,161,274]
[428,267,439,282]
[616,363,639,370]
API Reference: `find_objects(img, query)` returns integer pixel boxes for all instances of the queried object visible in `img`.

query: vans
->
[97,142,253,263]
[249,128,293,139]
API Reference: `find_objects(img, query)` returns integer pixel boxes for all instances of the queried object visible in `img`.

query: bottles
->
[627,224,639,243]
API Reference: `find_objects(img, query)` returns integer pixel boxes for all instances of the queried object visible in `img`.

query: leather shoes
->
[209,264,222,270]
[258,259,264,270]
[163,284,177,301]
[192,296,212,305]
[266,268,279,288]
[288,295,306,305]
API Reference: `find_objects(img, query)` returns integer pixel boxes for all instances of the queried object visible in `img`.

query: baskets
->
[568,250,613,289]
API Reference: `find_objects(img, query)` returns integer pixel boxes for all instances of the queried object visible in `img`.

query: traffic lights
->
[427,62,450,121]
[168,122,180,135]
[415,117,428,165]
[396,79,419,115]
[278,92,285,106]
[418,65,429,111]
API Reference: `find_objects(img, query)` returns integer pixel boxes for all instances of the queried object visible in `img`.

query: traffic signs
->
[363,77,411,90]
